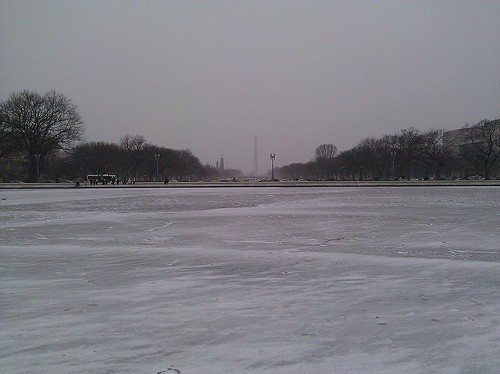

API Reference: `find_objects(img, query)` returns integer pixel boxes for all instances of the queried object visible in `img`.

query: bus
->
[87,174,117,182]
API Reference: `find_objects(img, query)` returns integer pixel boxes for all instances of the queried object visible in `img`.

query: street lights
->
[391,149,396,180]
[155,153,161,181]
[270,153,275,179]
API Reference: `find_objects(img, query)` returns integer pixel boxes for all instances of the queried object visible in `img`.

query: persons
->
[76,176,137,188]
